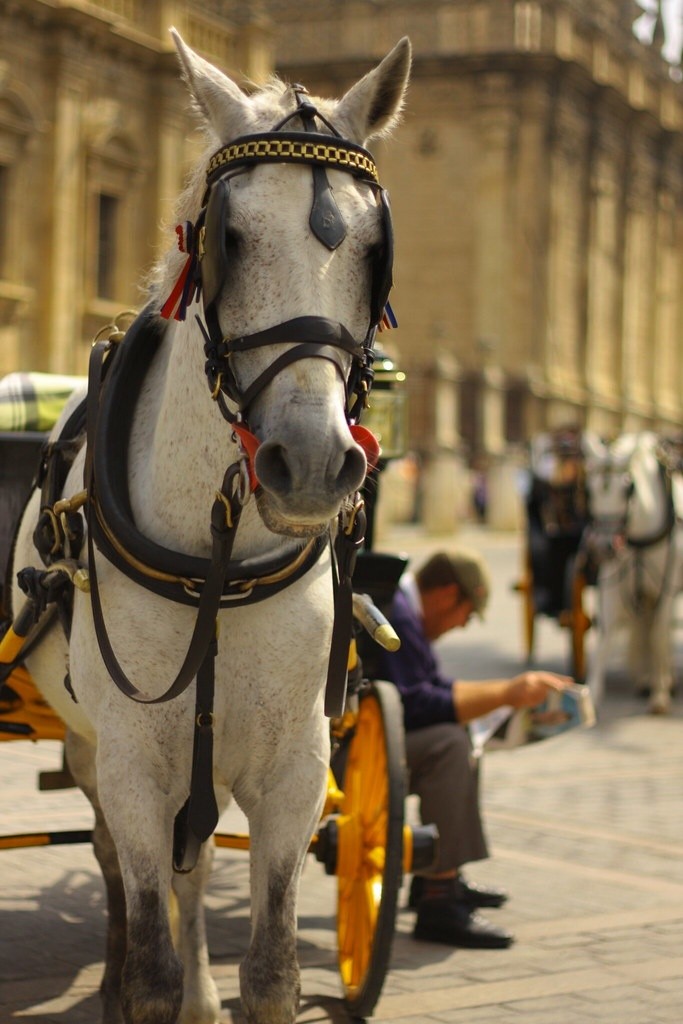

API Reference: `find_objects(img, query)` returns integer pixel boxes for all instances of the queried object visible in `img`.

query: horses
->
[580,428,683,715]
[11,23,412,1024]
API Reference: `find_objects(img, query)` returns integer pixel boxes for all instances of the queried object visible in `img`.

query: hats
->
[445,551,490,623]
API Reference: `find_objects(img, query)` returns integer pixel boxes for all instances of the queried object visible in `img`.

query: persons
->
[528,430,603,616]
[530,708,571,728]
[330,549,575,947]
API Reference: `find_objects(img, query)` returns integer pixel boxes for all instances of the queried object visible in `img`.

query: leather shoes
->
[414,901,515,946]
[409,875,507,906]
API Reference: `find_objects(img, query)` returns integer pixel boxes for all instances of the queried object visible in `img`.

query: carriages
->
[1,27,443,1024]
